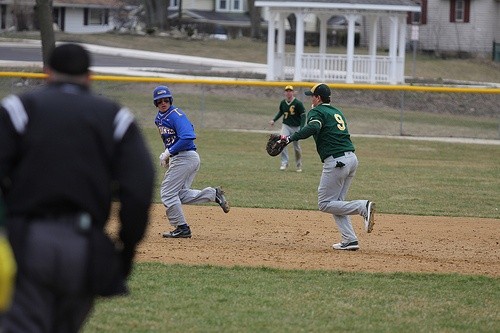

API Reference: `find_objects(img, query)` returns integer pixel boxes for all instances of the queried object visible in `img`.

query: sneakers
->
[333,241,359,250]
[296,168,302,173]
[280,164,288,171]
[364,201,376,233]
[215,186,230,213]
[163,225,191,238]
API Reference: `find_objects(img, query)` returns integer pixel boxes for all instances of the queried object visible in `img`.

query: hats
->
[48,44,91,74]
[284,85,293,91]
[153,86,172,101]
[304,83,331,98]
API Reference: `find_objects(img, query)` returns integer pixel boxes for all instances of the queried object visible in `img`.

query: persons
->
[153,85,230,238]
[0,44,156,333]
[266,84,377,249]
[270,85,306,172]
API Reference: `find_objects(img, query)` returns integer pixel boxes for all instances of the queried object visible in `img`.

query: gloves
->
[159,148,171,166]
[121,247,136,278]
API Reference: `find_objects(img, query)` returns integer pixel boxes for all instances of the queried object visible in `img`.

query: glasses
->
[156,100,169,104]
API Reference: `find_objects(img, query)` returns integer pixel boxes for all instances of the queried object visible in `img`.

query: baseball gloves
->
[265,134,288,157]
